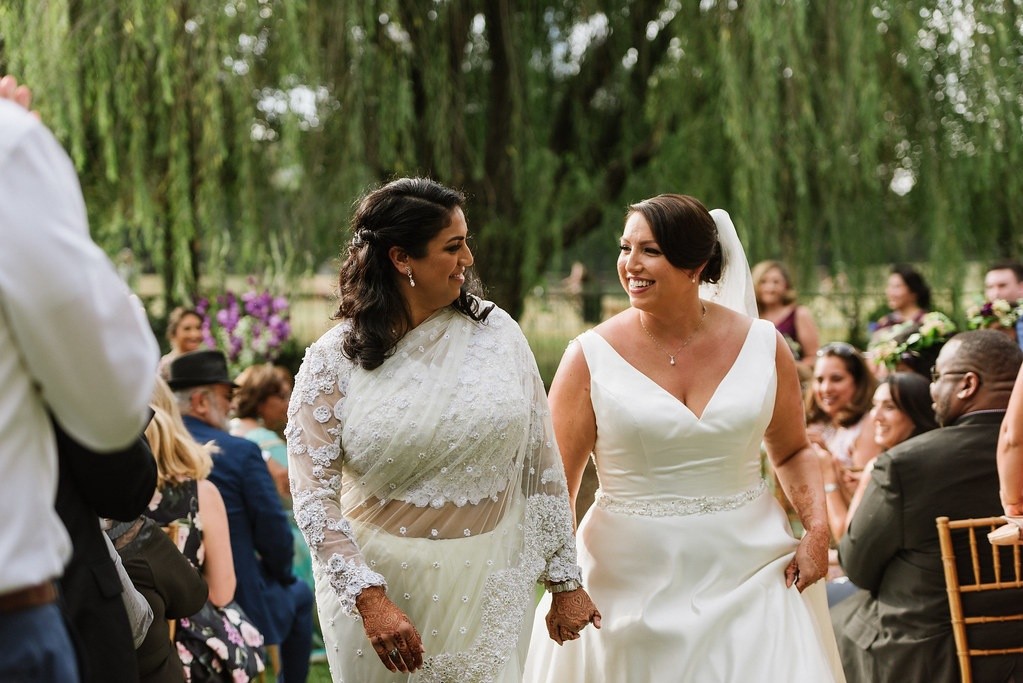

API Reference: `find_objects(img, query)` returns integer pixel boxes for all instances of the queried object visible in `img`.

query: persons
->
[751,260,821,367]
[873,263,931,330]
[521,194,846,683]
[228,361,315,593]
[995,361,1023,518]
[797,341,940,608]
[830,328,1023,683]
[166,350,314,683]
[285,178,601,683]
[0,75,160,683]
[47,374,263,683]
[984,260,1023,302]
[158,307,205,380]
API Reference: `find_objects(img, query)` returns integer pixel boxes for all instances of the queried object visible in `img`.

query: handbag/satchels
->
[99,528,155,651]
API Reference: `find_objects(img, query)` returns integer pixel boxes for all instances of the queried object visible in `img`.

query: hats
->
[165,349,242,389]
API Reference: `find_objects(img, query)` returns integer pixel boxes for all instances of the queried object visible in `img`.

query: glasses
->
[813,343,863,362]
[216,392,235,401]
[929,365,983,387]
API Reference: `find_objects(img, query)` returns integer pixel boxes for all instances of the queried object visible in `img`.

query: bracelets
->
[824,483,840,492]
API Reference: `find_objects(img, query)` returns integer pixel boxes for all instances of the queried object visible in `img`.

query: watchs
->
[549,579,580,593]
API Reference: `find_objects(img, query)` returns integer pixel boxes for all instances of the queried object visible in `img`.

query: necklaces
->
[641,300,706,365]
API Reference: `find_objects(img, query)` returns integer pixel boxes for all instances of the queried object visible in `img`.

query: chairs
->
[936,517,1023,683]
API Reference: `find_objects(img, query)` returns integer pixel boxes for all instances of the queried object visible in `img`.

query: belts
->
[0,579,60,612]
